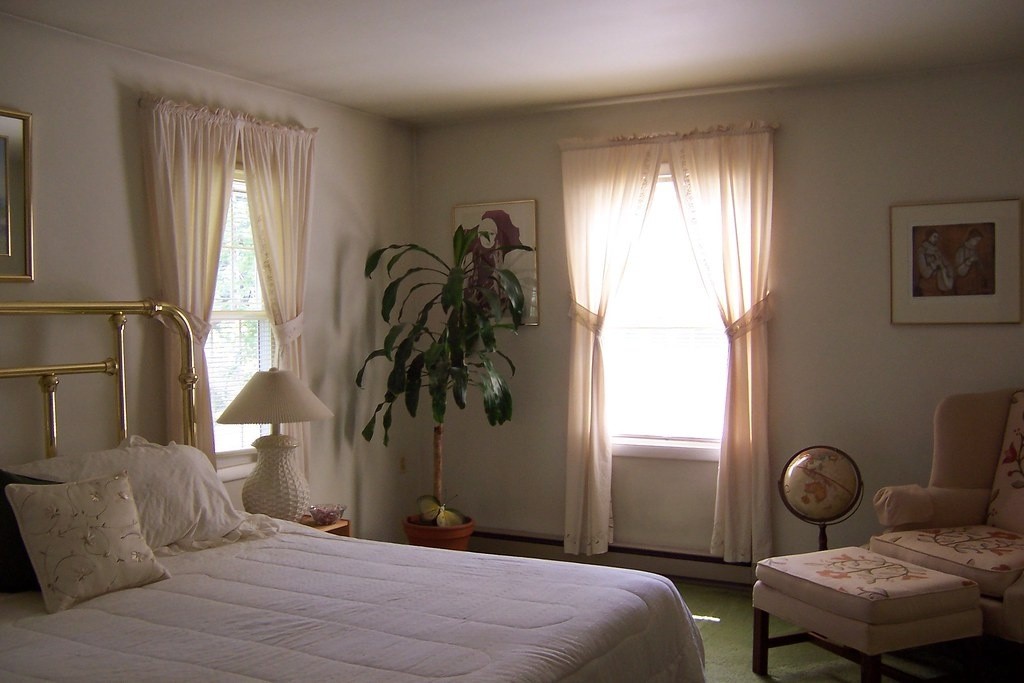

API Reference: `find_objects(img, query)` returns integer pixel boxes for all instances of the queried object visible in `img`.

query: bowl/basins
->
[308,503,347,525]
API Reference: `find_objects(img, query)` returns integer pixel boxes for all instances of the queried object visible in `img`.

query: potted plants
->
[354,221,535,552]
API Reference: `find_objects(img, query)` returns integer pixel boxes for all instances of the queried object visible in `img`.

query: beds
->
[0,303,708,681]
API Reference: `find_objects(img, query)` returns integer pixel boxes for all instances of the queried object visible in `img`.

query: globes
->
[778,445,865,552]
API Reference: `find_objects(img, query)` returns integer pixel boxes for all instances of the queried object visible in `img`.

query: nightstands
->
[299,513,352,541]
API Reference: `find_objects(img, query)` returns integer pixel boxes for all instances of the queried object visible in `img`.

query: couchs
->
[864,385,1023,646]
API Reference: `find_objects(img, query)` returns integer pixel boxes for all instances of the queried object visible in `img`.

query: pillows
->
[5,470,170,614]
[6,444,278,550]
[0,470,66,594]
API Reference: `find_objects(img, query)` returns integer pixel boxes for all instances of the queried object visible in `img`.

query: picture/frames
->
[890,198,1022,325]
[0,109,37,282]
[453,197,539,327]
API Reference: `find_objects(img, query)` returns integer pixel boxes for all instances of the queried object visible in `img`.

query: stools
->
[752,549,985,683]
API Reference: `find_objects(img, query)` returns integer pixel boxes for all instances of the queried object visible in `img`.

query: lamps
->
[216,366,336,522]
